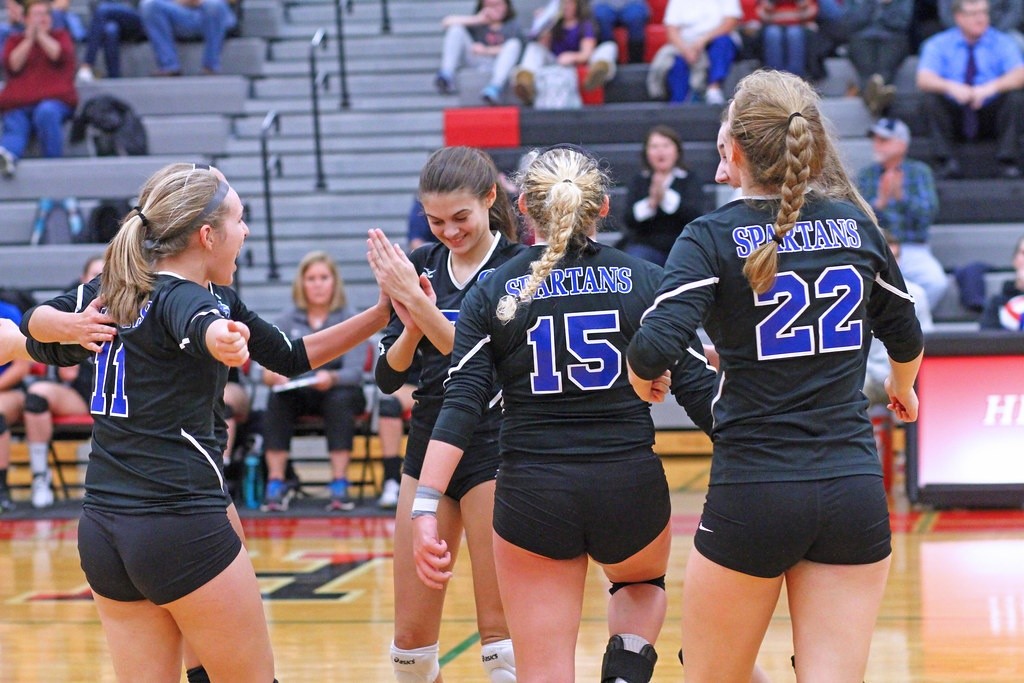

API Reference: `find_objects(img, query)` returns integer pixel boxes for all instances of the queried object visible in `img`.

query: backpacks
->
[68,94,148,158]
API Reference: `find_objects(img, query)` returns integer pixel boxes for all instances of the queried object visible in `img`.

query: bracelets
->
[410,485,444,520]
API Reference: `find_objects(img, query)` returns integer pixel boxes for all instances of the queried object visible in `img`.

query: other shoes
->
[147,68,179,76]
[0,484,11,511]
[514,71,535,105]
[76,66,95,81]
[865,74,898,117]
[582,60,609,92]
[31,472,54,507]
[435,68,460,95]
[705,86,726,106]
[0,145,17,180]
[482,83,506,105]
[944,160,963,179]
[997,163,1023,178]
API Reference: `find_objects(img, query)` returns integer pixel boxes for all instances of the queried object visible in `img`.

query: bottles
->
[63,194,83,235]
[243,455,264,509]
[30,194,55,246]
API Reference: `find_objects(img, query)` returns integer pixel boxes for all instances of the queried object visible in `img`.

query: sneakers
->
[324,490,356,512]
[260,479,296,514]
[379,478,400,508]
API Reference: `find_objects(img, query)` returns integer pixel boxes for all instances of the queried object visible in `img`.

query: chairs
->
[8,340,412,504]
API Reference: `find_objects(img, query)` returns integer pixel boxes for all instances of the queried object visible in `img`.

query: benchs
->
[0,0,1024,347]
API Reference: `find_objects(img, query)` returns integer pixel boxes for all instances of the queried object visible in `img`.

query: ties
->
[964,47,978,139]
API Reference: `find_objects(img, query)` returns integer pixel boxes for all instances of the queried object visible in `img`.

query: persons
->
[365,145,530,683]
[436,0,1024,114]
[976,237,1024,331]
[851,117,950,406]
[0,0,79,177]
[611,124,707,269]
[0,168,279,683]
[411,145,718,683]
[626,68,924,683]
[914,0,1024,180]
[19,162,391,683]
[0,0,240,82]
[406,193,441,251]
[677,97,795,683]
[0,250,419,510]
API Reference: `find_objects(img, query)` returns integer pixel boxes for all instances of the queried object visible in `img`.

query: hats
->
[867,117,911,146]
